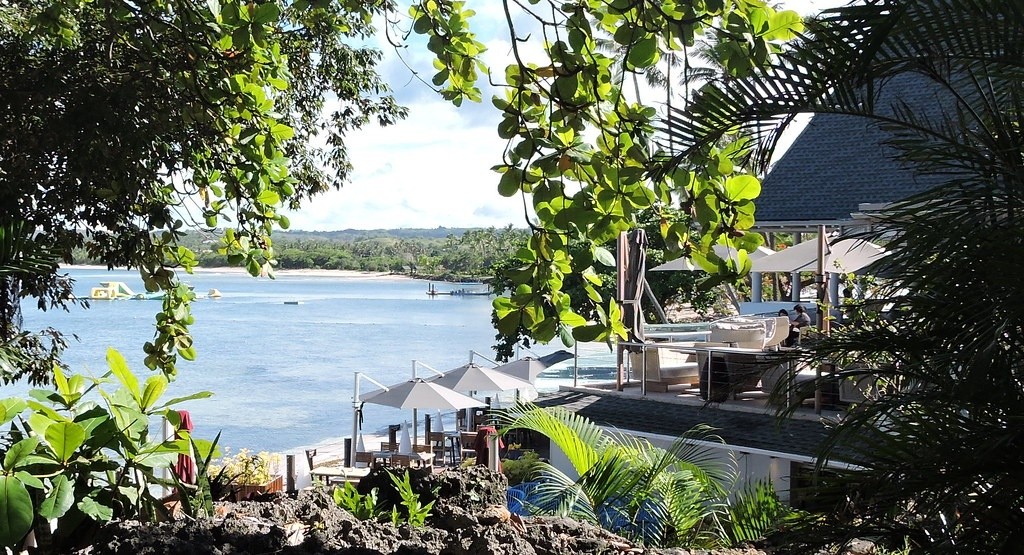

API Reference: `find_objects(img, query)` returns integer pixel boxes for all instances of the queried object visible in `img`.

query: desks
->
[437,431,460,465]
[644,322,710,331]
[368,450,436,467]
[310,467,370,485]
[704,347,802,408]
[645,331,712,342]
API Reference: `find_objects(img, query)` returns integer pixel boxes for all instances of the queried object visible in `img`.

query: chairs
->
[330,409,508,487]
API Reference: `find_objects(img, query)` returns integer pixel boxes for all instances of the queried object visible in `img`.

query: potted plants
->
[208,446,283,503]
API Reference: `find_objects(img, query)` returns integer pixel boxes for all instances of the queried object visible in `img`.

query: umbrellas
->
[353,350,578,411]
[649,232,899,275]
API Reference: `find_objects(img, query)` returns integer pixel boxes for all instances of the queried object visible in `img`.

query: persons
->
[474,415,504,475]
[843,279,862,318]
[778,304,811,347]
[783,281,792,301]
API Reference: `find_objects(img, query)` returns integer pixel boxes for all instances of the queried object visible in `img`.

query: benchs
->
[629,311,843,392]
